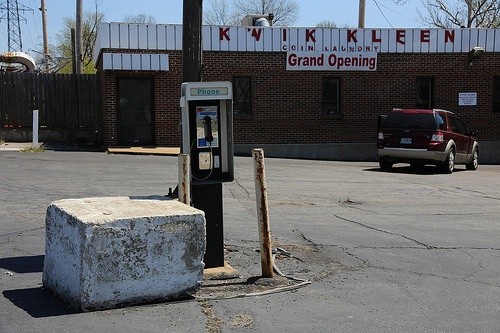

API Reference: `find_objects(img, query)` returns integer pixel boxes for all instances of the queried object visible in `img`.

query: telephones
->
[202,116,213,146]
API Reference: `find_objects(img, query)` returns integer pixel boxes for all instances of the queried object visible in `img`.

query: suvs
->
[378,106,479,173]
